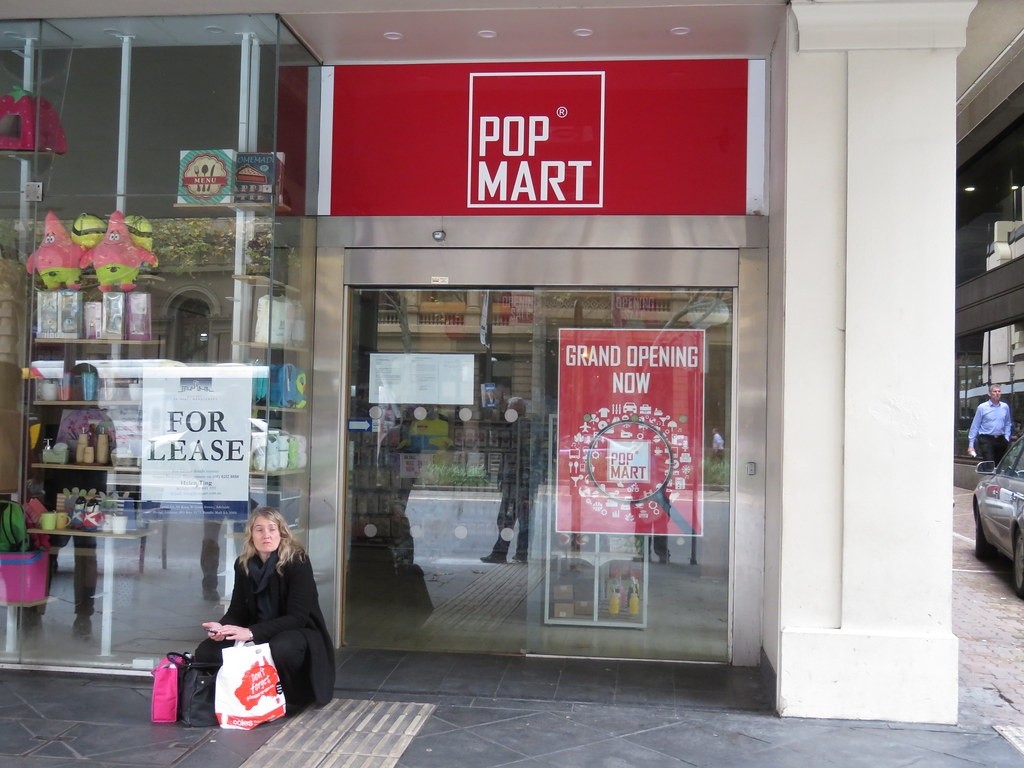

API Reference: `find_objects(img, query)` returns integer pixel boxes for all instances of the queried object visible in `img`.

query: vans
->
[31,359,219,445]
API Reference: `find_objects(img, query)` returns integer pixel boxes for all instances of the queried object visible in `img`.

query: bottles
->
[77,432,88,462]
[626,583,640,615]
[608,588,621,615]
[97,434,109,463]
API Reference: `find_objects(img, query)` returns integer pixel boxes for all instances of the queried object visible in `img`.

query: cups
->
[101,513,112,532]
[81,372,96,401]
[84,446,94,463]
[39,383,57,401]
[38,513,56,530]
[112,516,128,535]
[56,512,72,529]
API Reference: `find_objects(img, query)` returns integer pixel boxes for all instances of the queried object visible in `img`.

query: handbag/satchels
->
[150,654,183,723]
[215,639,287,730]
[167,650,219,727]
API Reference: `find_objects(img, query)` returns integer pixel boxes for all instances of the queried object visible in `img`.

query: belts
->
[981,433,1003,438]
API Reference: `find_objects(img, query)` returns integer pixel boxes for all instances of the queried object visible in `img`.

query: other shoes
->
[479,553,508,564]
[658,557,667,563]
[18,609,42,631]
[72,613,91,635]
[633,556,651,562]
[510,554,526,564]
[202,588,221,601]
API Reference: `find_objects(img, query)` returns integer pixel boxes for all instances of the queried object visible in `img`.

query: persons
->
[480,397,541,564]
[179,411,253,601]
[712,428,724,462]
[380,410,422,510]
[629,444,671,563]
[195,507,335,712]
[26,363,117,635]
[968,384,1012,468]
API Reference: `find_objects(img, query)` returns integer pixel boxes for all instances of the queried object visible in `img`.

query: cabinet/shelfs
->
[25,337,165,655]
[350,415,539,577]
[543,414,648,629]
[231,274,307,477]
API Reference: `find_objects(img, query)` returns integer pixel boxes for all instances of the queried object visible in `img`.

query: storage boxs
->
[38,291,154,341]
[177,150,287,204]
[0,550,50,602]
[552,579,593,618]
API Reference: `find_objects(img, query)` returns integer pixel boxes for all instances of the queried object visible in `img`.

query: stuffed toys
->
[27,210,86,291]
[78,211,159,292]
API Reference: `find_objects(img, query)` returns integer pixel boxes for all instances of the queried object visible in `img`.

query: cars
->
[146,416,307,473]
[541,434,656,489]
[972,430,1023,599]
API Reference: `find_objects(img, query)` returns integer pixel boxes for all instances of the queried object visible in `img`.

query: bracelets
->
[249,631,254,640]
